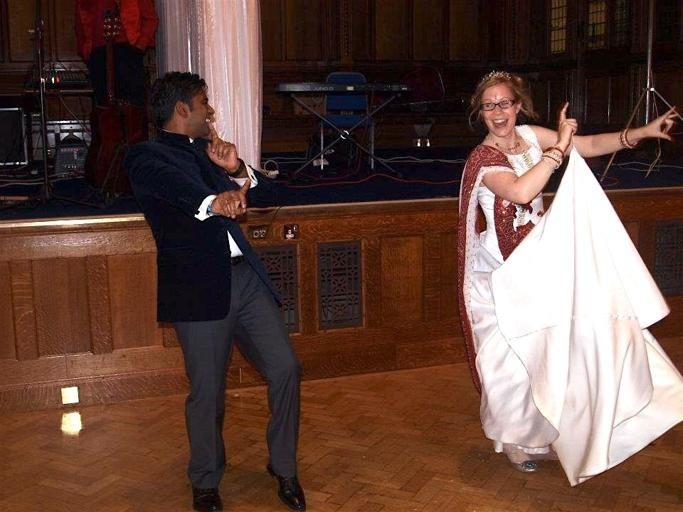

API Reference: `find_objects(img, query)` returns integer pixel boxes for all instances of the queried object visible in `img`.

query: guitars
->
[85,15,148,195]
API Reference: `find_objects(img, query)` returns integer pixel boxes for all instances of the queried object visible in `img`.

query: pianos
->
[278,80,408,95]
[39,61,89,86]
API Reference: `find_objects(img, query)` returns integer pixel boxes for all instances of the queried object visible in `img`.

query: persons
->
[455,68,678,473]
[125,70,307,512]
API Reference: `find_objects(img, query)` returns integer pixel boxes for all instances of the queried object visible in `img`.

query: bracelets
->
[619,124,636,151]
[227,158,245,178]
[541,143,564,173]
[208,197,220,216]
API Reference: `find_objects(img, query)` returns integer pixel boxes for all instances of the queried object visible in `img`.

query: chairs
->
[318,71,375,170]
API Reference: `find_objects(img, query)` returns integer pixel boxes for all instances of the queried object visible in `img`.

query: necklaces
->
[482,132,524,154]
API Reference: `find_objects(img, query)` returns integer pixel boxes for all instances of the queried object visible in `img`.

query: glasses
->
[480,100,514,110]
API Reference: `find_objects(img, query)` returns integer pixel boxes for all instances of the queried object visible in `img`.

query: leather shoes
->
[512,461,538,472]
[192,486,223,512]
[266,464,306,511]
